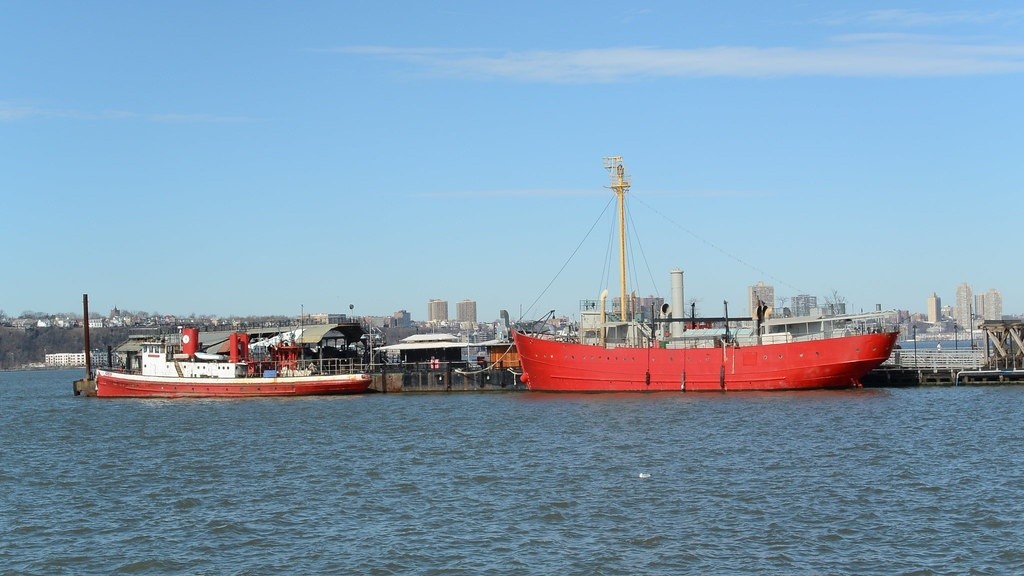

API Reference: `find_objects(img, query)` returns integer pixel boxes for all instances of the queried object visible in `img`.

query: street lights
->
[912,325,917,369]
[953,318,958,354]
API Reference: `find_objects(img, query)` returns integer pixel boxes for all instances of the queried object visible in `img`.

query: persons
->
[937,342,943,353]
[381,352,390,364]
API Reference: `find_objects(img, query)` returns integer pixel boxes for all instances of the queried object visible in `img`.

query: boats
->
[94,326,372,398]
[511,156,902,391]
